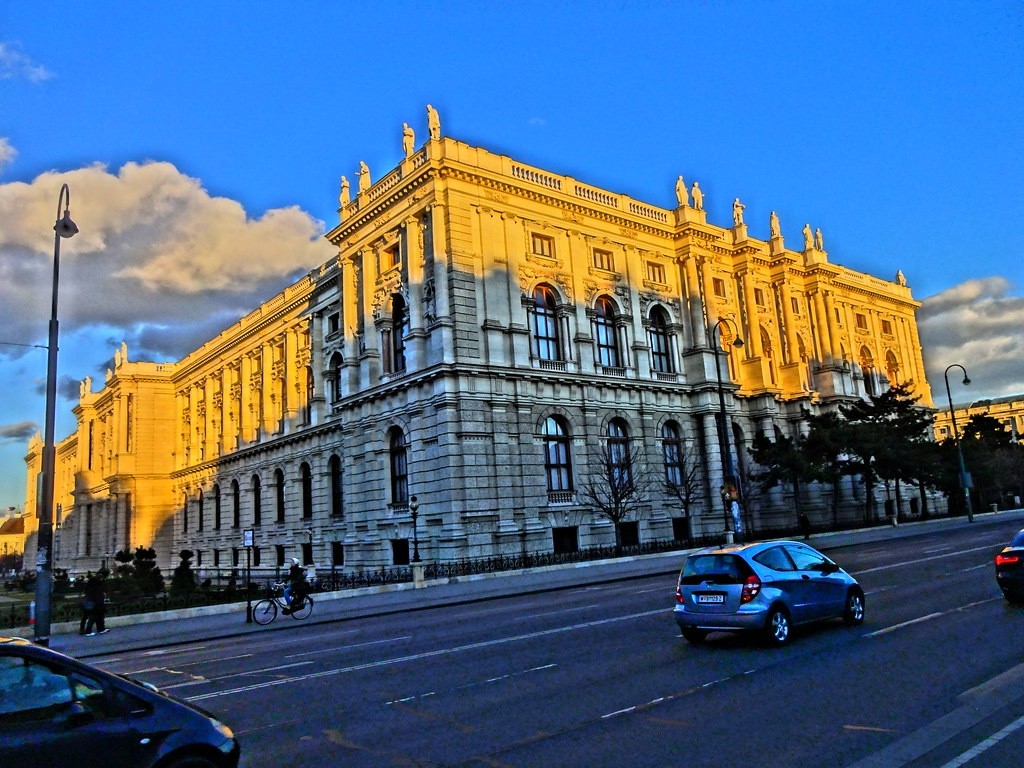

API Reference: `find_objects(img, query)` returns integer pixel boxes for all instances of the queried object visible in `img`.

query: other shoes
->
[85,632,97,637]
[281,605,291,609]
[100,629,110,634]
[79,629,84,635]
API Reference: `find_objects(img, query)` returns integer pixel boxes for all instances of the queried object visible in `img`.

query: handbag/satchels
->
[303,582,310,593]
[83,599,96,610]
[80,592,88,603]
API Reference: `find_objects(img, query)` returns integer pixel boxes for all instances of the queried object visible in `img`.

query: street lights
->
[712,317,745,546]
[944,363,976,525]
[31,182,80,647]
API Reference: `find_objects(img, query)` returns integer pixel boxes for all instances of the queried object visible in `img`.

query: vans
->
[0,637,240,768]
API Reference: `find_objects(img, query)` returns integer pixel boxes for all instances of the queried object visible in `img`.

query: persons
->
[800,513,810,540]
[282,559,305,604]
[80,572,110,637]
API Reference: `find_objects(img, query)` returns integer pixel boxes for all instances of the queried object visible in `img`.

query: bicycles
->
[253,582,313,626]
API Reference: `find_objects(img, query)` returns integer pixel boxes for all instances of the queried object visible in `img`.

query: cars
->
[672,539,865,644]
[993,527,1024,605]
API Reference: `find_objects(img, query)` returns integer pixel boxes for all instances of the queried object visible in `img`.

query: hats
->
[292,558,299,564]
[97,568,109,577]
[95,574,103,581]
